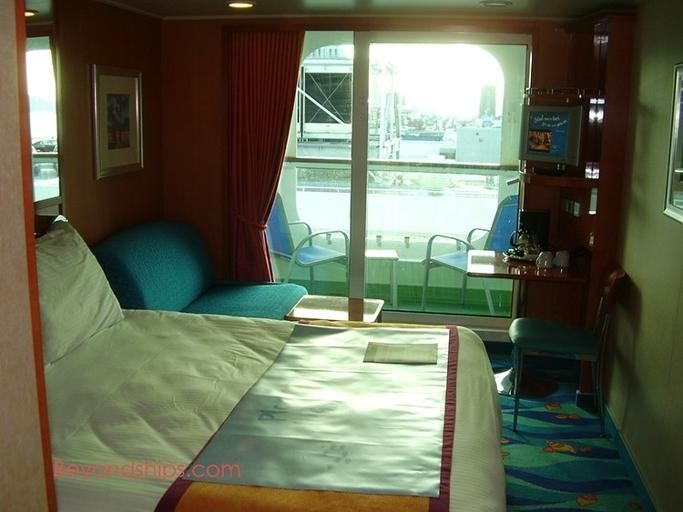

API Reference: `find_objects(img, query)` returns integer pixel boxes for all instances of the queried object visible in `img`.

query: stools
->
[364,249,400,309]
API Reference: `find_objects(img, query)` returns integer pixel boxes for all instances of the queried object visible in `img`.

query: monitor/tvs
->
[518,103,583,167]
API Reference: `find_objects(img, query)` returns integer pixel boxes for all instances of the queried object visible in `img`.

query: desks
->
[284,294,384,323]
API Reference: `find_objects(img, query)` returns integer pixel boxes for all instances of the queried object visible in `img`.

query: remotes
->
[533,167,565,175]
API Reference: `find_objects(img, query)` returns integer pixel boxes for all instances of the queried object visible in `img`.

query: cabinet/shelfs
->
[466,9,637,408]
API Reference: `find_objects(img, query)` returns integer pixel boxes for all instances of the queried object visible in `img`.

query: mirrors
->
[663,63,683,223]
[24,22,66,214]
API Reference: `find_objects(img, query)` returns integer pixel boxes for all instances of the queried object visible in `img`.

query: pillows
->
[35,215,125,365]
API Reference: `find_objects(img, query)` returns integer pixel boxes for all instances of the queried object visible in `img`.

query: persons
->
[111,95,125,127]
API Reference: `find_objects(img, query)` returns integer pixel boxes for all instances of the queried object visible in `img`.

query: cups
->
[536,251,553,269]
[552,248,571,267]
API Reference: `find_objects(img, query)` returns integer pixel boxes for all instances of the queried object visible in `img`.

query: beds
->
[43,308,507,512]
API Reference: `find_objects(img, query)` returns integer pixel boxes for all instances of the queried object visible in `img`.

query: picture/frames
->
[94,63,144,180]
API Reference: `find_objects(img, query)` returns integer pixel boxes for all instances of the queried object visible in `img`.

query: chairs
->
[420,194,519,316]
[89,216,308,320]
[507,269,627,437]
[263,191,350,297]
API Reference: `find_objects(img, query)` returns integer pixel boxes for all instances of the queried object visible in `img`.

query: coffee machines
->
[502,208,551,262]
